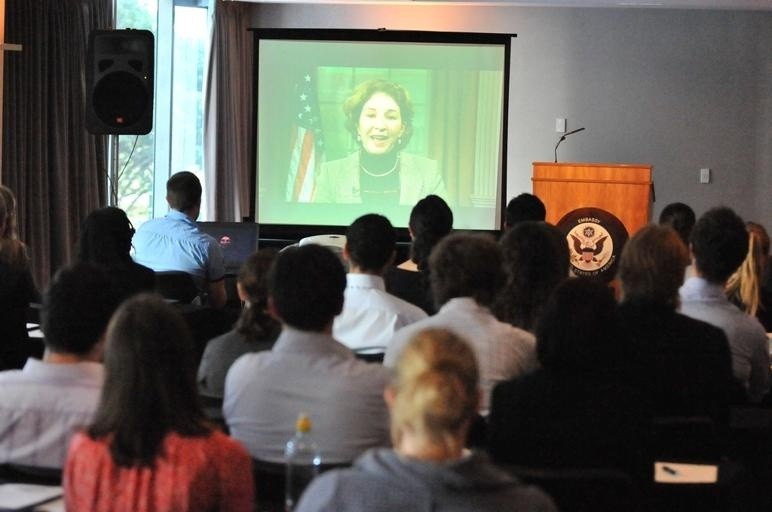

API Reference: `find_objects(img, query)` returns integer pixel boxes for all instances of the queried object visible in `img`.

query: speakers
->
[84,28,155,135]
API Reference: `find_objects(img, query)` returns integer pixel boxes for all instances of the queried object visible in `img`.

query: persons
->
[312,77,449,205]
[0,171,771,512]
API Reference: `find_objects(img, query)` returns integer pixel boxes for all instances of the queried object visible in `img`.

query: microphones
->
[554,127,585,163]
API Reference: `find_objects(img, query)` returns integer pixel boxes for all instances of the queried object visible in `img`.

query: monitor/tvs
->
[195,221,260,269]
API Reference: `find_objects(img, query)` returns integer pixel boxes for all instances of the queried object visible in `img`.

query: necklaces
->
[359,155,399,177]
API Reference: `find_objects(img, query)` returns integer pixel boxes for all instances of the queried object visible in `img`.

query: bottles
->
[285,412,322,511]
